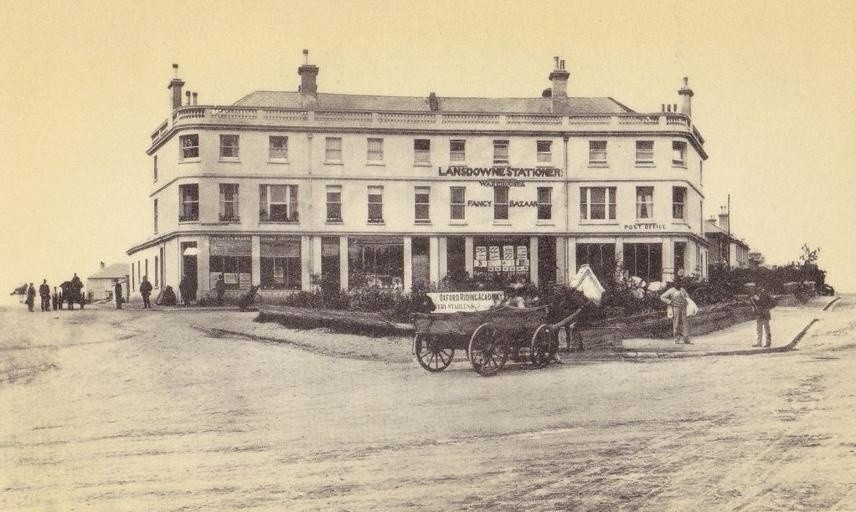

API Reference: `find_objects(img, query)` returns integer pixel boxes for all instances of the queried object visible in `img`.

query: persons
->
[179,271,196,307]
[52,286,58,310]
[660,279,694,344]
[215,274,226,303]
[113,278,123,309]
[26,282,35,312]
[394,278,403,290]
[415,284,436,315]
[749,286,776,348]
[140,275,152,309]
[58,292,64,309]
[38,279,50,312]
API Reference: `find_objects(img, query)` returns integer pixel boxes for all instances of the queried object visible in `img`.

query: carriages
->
[51,280,84,310]
[407,284,593,377]
